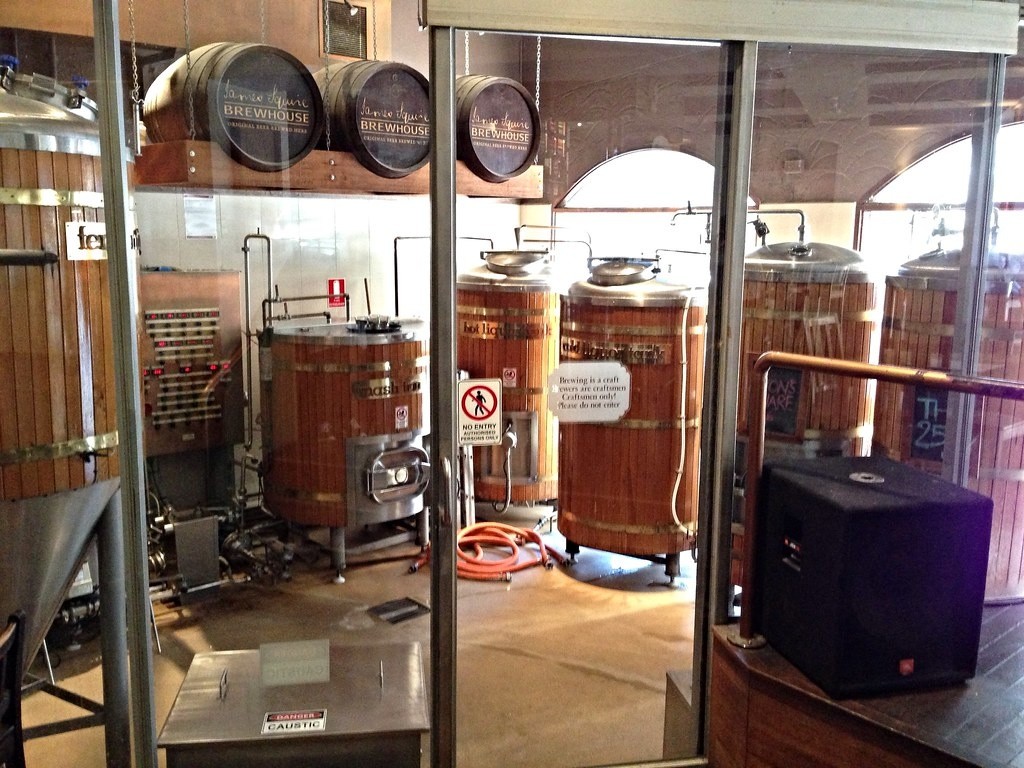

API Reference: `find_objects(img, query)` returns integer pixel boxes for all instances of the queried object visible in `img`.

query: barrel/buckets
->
[309,59,431,178]
[455,74,542,183]
[143,41,324,172]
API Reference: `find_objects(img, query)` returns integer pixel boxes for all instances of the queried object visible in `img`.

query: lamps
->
[344,0,358,16]
